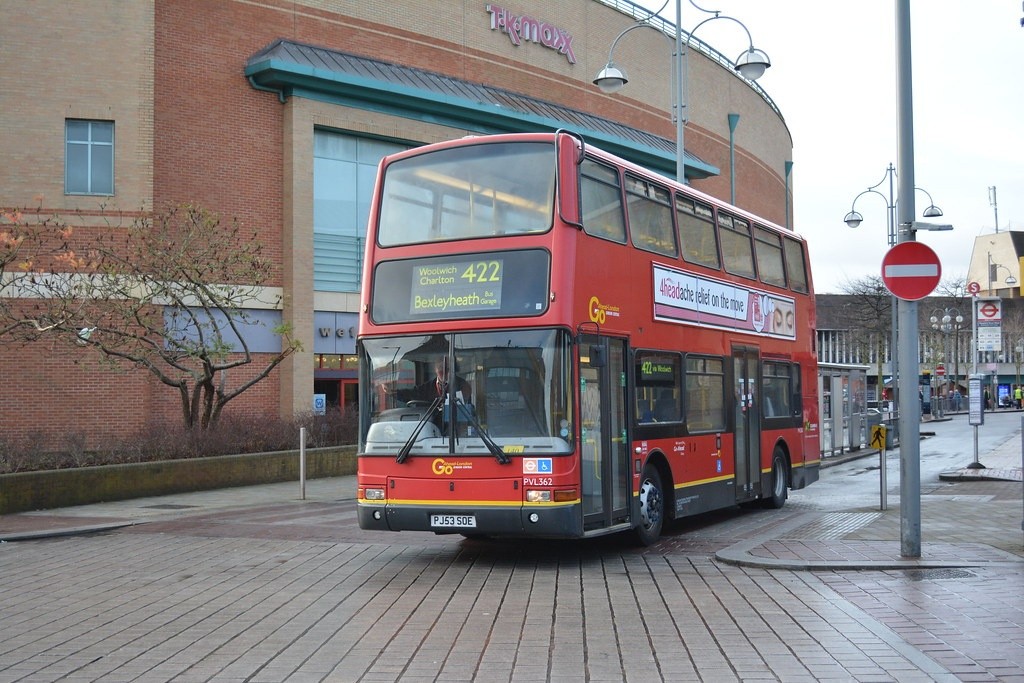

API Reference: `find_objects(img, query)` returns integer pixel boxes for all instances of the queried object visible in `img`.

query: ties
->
[440,383,447,397]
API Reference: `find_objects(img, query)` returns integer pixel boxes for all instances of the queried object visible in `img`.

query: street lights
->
[841,163,945,442]
[928,306,1023,425]
[591,0,773,185]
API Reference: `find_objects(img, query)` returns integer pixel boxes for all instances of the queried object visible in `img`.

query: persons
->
[381,355,472,437]
[983,387,992,409]
[1013,386,1023,409]
[953,389,962,411]
[948,392,954,409]
[772,308,794,334]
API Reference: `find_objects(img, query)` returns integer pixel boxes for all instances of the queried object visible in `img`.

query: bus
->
[355,130,819,547]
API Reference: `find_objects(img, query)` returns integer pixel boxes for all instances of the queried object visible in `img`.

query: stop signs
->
[936,366,944,375]
[882,240,942,300]
